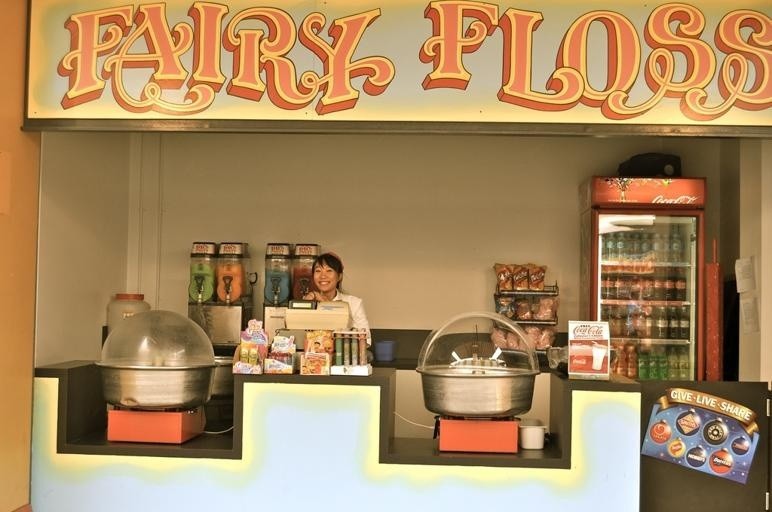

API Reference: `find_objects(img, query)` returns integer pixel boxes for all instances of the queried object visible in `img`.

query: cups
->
[590,345,607,372]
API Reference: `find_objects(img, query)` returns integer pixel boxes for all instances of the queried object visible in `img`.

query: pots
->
[414,367,542,421]
[93,361,214,413]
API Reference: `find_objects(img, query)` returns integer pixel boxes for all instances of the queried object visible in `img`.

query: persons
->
[303,252,372,354]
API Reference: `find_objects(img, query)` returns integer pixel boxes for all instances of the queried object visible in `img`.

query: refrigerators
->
[576,177,722,381]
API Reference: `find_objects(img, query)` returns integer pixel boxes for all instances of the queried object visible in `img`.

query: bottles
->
[681,307,690,339]
[351,337,358,365]
[616,276,623,299]
[631,276,640,299]
[672,235,684,262]
[602,308,611,336]
[617,232,627,260]
[642,235,652,260]
[617,345,628,377]
[358,334,366,365]
[634,235,643,261]
[669,307,678,338]
[606,234,614,258]
[336,339,343,364]
[653,235,663,260]
[622,313,635,337]
[665,268,674,300]
[639,345,651,381]
[623,276,630,299]
[603,275,616,299]
[627,347,638,379]
[649,347,660,381]
[680,347,691,381]
[646,313,654,339]
[675,268,686,300]
[626,233,635,260]
[643,274,653,300]
[657,307,669,339]
[659,345,669,381]
[653,267,663,300]
[668,347,681,381]
[609,346,617,376]
[636,314,646,339]
[601,236,606,258]
[343,340,350,365]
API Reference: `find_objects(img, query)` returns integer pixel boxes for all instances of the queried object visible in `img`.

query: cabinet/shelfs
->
[495,280,563,369]
